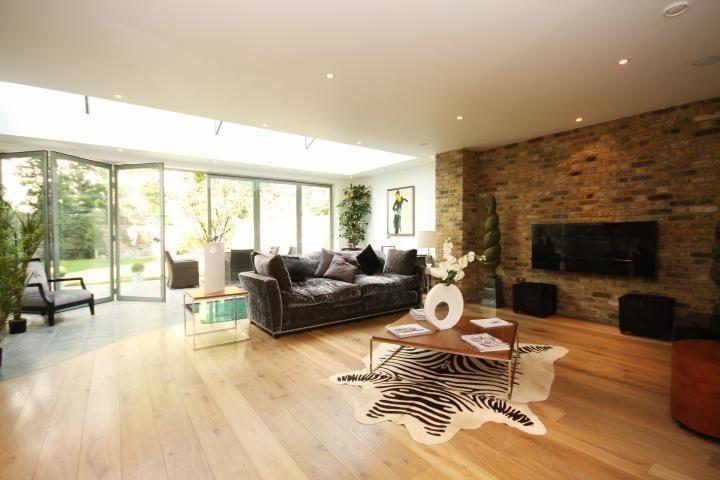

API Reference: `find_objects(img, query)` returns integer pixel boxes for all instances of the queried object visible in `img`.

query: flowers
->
[424,236,487,287]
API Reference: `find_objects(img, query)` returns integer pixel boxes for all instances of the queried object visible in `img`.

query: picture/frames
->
[384,185,415,237]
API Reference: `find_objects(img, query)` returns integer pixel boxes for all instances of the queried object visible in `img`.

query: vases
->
[422,283,465,332]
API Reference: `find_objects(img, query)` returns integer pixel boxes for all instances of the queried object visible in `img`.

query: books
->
[469,314,514,329]
[409,307,428,322]
[387,321,432,340]
[459,332,511,354]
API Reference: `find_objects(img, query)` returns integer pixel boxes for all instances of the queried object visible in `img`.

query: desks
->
[370,306,522,401]
[180,285,253,351]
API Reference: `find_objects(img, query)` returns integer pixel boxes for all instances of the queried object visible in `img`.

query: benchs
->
[8,255,98,328]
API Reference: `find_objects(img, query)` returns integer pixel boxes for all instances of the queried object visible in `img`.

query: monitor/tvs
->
[531,221,659,279]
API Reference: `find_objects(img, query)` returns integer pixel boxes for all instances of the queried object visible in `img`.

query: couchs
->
[239,246,434,340]
[224,245,257,286]
[164,248,203,289]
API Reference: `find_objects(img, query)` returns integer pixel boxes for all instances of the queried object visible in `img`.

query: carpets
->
[331,339,571,447]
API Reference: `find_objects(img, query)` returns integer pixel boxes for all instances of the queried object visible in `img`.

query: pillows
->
[314,247,349,278]
[279,256,307,283]
[323,254,359,284]
[382,247,418,276]
[356,243,384,277]
[253,255,293,292]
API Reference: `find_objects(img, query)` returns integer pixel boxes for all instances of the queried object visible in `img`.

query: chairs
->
[288,246,296,254]
[268,246,282,254]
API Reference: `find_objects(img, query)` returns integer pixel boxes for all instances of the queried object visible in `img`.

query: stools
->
[669,335,720,442]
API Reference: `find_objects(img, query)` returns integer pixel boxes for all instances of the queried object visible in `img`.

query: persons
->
[390,190,410,233]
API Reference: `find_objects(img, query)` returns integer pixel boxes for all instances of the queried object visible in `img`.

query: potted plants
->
[185,209,234,295]
[334,183,374,252]
[3,207,54,337]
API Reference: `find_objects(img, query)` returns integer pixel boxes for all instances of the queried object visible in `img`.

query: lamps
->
[418,231,438,264]
